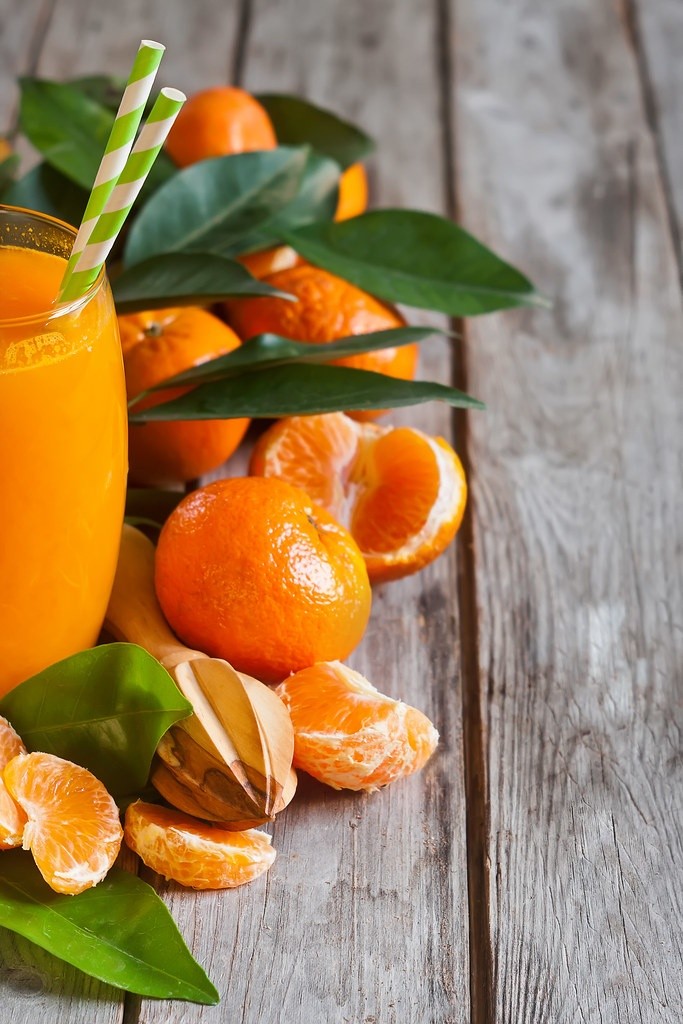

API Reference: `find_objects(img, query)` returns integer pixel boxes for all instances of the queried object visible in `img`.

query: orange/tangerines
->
[0,160,469,891]
[165,87,278,169]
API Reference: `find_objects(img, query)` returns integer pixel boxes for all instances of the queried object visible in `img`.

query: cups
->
[1,204,128,696]
